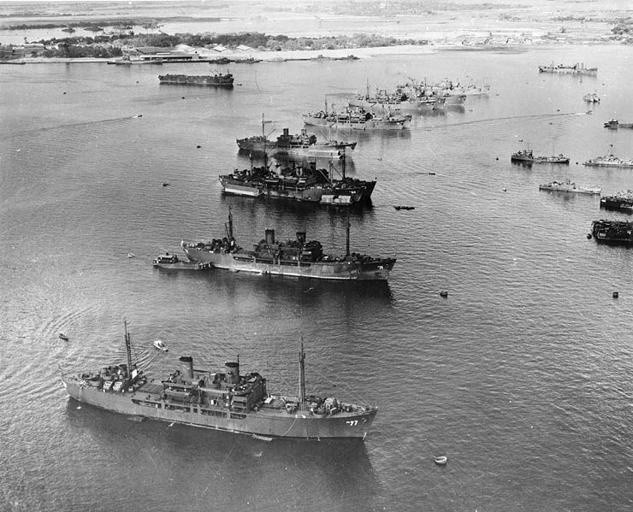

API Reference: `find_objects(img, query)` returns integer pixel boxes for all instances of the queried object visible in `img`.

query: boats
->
[510,118,633,244]
[152,206,396,281]
[158,73,234,89]
[538,62,597,74]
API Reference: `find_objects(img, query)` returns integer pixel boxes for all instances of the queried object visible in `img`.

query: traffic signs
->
[219,72,492,205]
